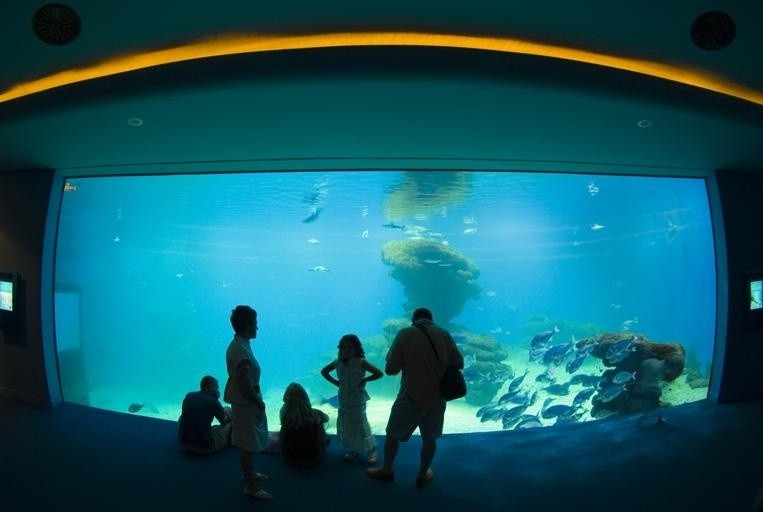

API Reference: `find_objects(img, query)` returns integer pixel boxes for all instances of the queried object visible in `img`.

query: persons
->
[367,307,463,489]
[321,334,383,466]
[223,305,274,502]
[279,383,330,471]
[174,376,232,457]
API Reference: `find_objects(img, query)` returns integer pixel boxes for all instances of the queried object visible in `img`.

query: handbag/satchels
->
[441,368,467,401]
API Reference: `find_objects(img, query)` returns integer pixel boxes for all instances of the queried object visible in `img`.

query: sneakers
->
[367,468,393,481]
[242,471,272,500]
[416,469,432,489]
[344,450,378,464]
